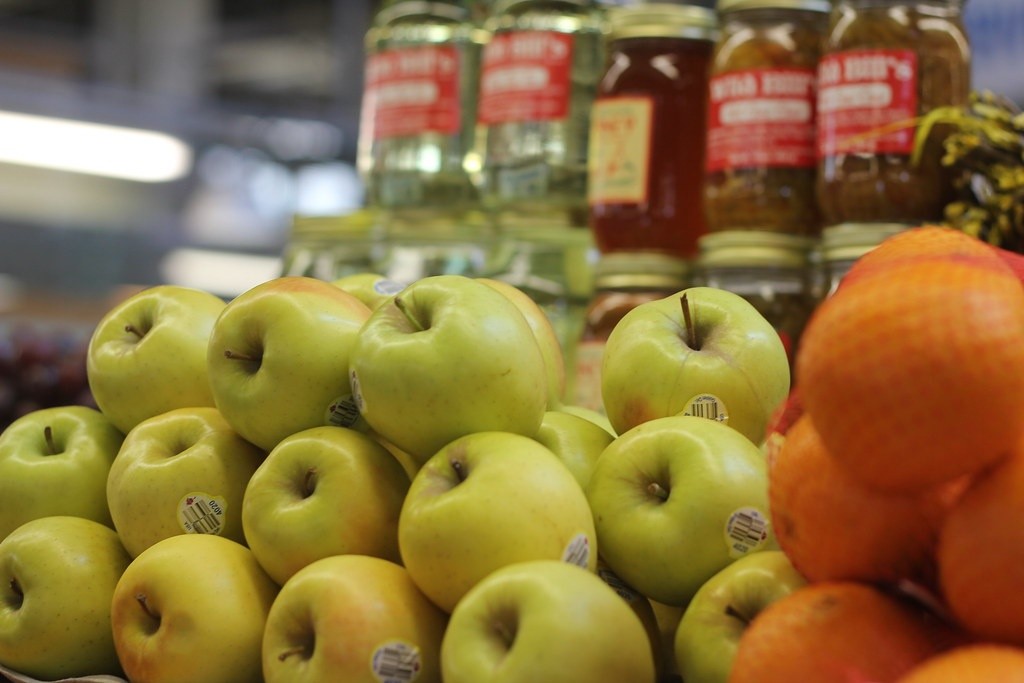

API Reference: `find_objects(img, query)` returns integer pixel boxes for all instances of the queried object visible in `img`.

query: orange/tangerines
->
[729,220,1024,683]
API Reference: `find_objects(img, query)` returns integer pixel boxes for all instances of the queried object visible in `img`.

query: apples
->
[0,269,805,683]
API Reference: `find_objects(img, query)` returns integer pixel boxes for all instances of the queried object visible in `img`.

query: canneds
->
[282,0,975,415]
